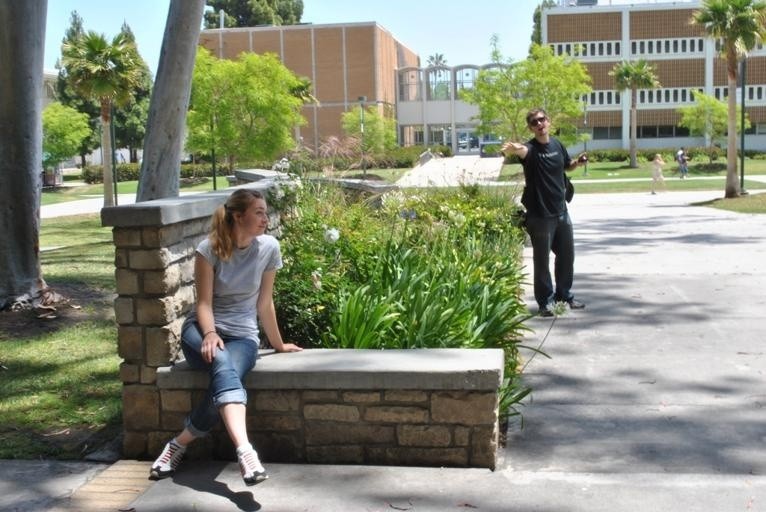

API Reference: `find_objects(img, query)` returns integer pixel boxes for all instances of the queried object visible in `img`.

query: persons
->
[150,190,303,485]
[501,108,589,316]
[651,153,666,195]
[675,147,689,180]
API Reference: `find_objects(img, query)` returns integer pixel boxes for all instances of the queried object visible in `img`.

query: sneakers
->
[235,445,269,486]
[651,190,656,194]
[554,297,586,310]
[148,439,186,480]
[538,301,553,316]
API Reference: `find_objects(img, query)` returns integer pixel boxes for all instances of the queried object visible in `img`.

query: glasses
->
[529,117,546,127]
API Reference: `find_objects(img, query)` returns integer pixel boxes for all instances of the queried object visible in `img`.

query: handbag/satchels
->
[564,176,575,204]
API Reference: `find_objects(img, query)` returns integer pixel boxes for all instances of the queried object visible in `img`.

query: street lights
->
[739,50,750,199]
[355,94,370,183]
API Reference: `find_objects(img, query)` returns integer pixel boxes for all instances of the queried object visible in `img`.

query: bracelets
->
[203,330,216,337]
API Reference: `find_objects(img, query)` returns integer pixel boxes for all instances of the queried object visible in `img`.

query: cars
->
[181,153,194,164]
[56,158,86,180]
[113,152,126,165]
[436,130,490,149]
